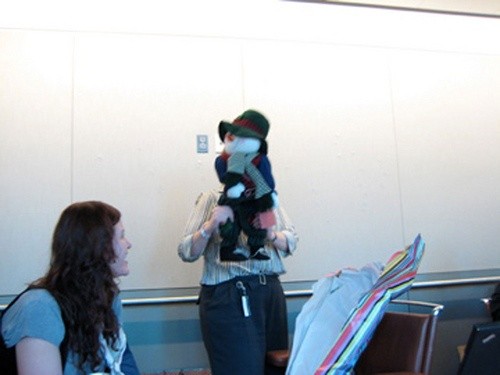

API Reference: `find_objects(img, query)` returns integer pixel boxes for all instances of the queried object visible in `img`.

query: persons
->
[177,143,298,374]
[0,200,140,375]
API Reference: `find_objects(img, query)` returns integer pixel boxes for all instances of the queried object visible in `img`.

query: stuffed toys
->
[215,109,277,261]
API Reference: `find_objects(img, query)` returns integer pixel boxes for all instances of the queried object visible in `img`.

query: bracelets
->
[200,224,211,240]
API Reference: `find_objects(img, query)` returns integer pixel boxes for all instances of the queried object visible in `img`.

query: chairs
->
[266,297,443,375]
